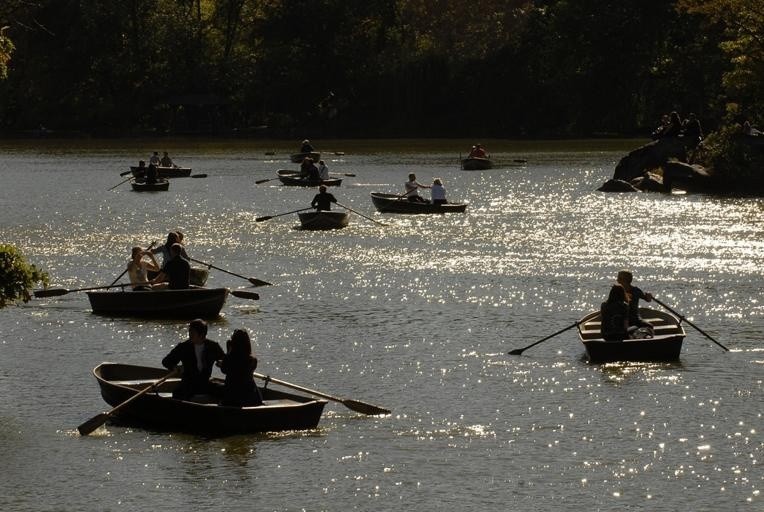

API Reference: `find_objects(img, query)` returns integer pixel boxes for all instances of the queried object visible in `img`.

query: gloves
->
[369,191,468,215]
[86,287,230,324]
[92,358,330,438]
[461,156,494,170]
[291,152,322,164]
[130,176,169,191]
[130,165,192,179]
[294,212,350,231]
[574,306,687,366]
[276,168,343,186]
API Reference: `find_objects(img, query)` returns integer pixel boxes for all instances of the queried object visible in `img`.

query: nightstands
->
[256,204,318,222]
[77,368,178,436]
[265,151,346,156]
[255,173,355,184]
[189,284,260,299]
[256,370,388,416]
[508,317,600,355]
[119,160,207,178]
[190,257,272,286]
[492,158,527,165]
[34,281,155,298]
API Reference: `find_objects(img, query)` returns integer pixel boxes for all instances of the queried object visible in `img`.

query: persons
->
[135,150,174,183]
[403,173,431,204]
[123,230,194,291]
[615,270,653,333]
[467,143,489,160]
[599,284,630,342]
[160,317,225,399]
[310,184,337,213]
[218,327,262,407]
[429,178,446,205]
[648,111,701,138]
[300,139,314,154]
[299,156,329,182]
